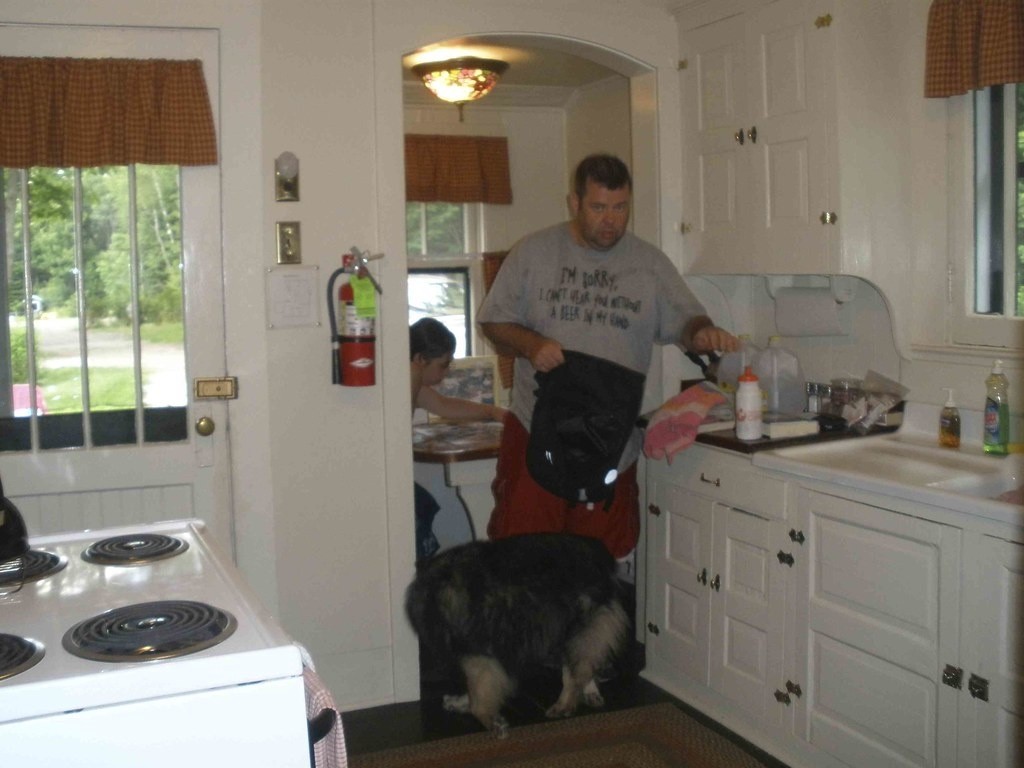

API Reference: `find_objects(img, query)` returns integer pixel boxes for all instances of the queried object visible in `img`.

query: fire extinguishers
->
[326,246,383,387]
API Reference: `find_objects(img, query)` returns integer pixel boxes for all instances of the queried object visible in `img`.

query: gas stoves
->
[0,518,315,767]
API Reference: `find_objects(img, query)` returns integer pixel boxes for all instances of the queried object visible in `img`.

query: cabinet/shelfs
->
[783,473,1023,767]
[639,433,796,765]
[612,448,647,668]
[681,2,950,277]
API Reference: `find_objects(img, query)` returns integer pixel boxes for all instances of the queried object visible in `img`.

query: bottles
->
[803,379,867,419]
[734,366,763,440]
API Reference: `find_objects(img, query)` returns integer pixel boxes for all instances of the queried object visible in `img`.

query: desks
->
[410,420,508,544]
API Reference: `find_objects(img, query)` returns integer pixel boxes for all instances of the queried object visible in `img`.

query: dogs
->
[403,531,631,739]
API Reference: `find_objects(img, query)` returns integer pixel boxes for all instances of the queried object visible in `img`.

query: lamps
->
[411,56,511,123]
[278,151,299,193]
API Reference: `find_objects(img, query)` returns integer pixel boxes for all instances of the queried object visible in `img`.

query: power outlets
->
[275,158,301,203]
[277,221,303,266]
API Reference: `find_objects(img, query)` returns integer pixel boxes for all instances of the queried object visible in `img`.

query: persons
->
[474,151,742,563]
[409,316,508,433]
